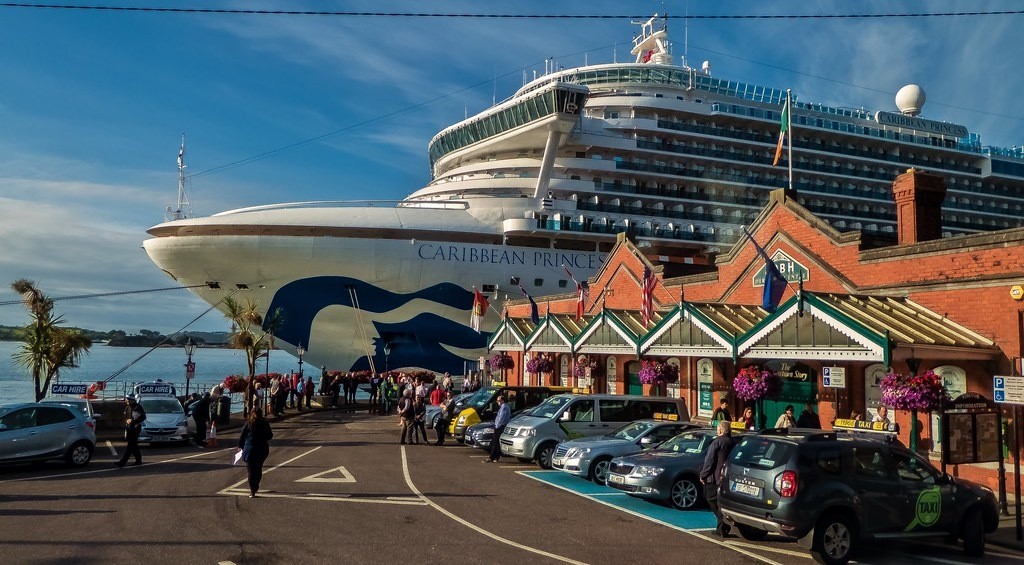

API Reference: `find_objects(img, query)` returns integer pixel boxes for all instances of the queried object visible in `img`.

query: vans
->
[446,387,552,443]
[499,393,690,470]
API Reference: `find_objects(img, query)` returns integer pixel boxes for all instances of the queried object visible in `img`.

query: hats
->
[126,393,135,400]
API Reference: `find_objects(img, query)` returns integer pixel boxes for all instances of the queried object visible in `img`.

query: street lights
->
[185,335,198,402]
[384,343,391,376]
[296,345,305,379]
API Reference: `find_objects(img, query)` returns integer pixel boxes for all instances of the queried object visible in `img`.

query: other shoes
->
[493,459,498,463]
[114,462,122,467]
[481,459,492,463]
[249,493,255,497]
[131,462,141,466]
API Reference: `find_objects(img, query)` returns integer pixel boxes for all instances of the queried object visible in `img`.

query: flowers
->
[734,366,769,399]
[638,359,668,383]
[880,370,952,414]
[330,370,434,384]
[526,356,552,374]
[574,360,596,376]
[490,354,511,370]
[224,373,280,388]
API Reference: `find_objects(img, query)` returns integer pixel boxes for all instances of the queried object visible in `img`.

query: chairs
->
[583,412,593,421]
[575,412,583,420]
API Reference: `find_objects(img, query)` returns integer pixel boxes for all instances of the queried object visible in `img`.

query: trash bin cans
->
[217,397,231,426]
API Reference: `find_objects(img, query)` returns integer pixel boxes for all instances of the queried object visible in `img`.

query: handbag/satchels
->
[188,400,200,410]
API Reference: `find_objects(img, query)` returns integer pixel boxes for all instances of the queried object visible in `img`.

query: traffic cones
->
[206,420,220,448]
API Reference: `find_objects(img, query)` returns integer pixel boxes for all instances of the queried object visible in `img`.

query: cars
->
[552,418,704,487]
[30,397,102,432]
[464,407,531,453]
[424,392,486,430]
[605,428,749,510]
[133,382,193,448]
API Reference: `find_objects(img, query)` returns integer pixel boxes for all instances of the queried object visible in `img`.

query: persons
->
[774,405,796,428]
[846,410,862,433]
[482,396,511,463]
[711,399,732,421]
[700,420,738,537]
[872,405,889,423]
[113,393,147,468]
[738,407,755,429]
[796,401,822,429]
[184,372,497,448]
[238,408,273,498]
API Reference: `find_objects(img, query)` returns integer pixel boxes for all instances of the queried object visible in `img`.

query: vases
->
[224,389,234,393]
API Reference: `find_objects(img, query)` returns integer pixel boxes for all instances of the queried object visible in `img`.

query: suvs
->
[0,403,97,471]
[718,426,1000,564]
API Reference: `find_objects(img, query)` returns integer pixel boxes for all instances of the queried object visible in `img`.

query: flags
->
[470,289,489,333]
[772,99,787,166]
[576,283,585,322]
[762,259,788,314]
[641,264,659,329]
[528,296,539,325]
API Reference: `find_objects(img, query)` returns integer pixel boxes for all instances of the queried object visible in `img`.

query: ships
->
[139,0,1024,390]
